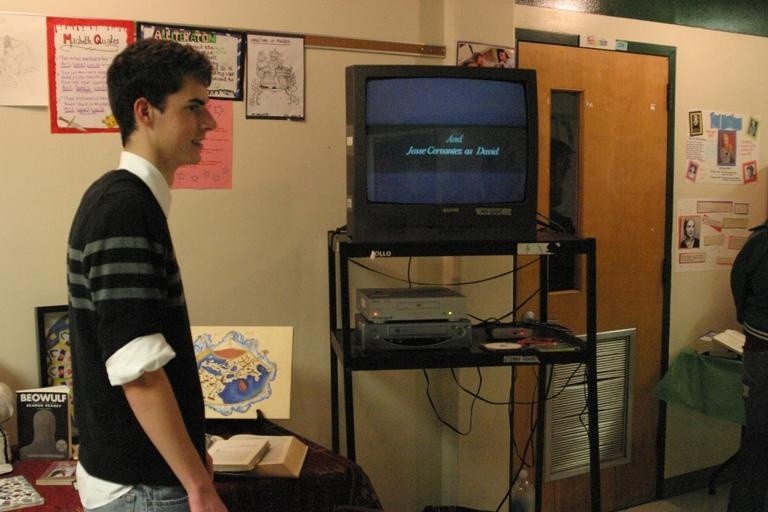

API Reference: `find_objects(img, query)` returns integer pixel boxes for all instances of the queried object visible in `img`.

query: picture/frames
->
[34,303,83,445]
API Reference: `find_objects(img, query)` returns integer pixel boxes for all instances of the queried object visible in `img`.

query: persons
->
[496,48,514,69]
[730,218,768,471]
[718,133,734,165]
[66,39,229,512]
[681,218,699,248]
[746,164,756,181]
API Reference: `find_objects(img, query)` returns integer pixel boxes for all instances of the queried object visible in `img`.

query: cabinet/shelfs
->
[326,228,602,510]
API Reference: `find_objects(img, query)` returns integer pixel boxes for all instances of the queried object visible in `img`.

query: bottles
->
[511,469,536,511]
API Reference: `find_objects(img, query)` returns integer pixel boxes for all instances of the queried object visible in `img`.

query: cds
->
[485,341,522,351]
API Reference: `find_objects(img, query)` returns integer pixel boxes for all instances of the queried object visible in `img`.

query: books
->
[35,460,77,485]
[16,385,70,460]
[208,432,309,478]
[696,329,746,358]
[0,474,44,512]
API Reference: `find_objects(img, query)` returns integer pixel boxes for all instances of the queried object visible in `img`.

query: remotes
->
[533,343,581,353]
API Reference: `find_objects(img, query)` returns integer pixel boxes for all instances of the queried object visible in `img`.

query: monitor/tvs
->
[345,63,539,245]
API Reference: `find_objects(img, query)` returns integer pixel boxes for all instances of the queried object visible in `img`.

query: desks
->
[0,416,360,512]
[677,348,748,494]
